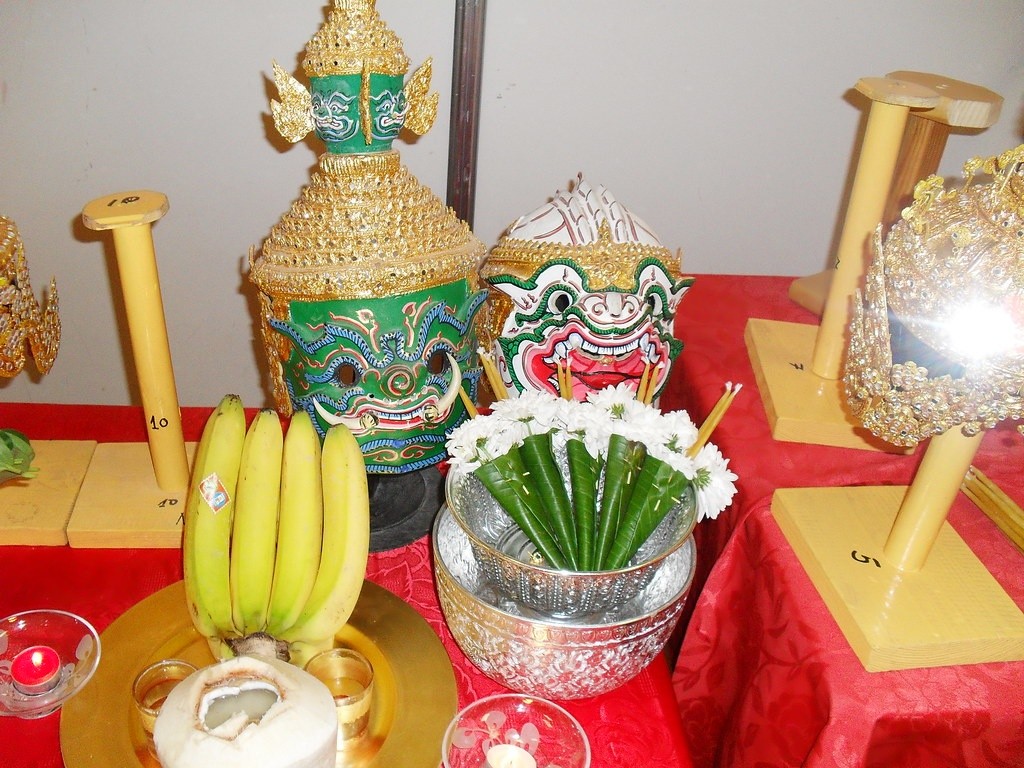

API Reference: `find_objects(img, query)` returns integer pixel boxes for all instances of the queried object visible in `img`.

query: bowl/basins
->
[447,444,700,620]
[422,502,699,703]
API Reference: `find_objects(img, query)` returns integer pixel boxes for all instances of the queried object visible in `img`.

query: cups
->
[304,648,373,751]
[132,660,201,763]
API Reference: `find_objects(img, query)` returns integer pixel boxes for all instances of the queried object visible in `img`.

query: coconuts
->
[153,654,339,768]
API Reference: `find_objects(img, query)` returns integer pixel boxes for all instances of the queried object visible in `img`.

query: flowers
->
[446,382,738,523]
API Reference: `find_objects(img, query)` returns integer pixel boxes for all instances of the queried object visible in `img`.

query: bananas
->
[183,394,370,663]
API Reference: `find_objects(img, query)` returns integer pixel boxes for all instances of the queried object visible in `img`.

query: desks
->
[663,274,1024,768]
[1,402,696,768]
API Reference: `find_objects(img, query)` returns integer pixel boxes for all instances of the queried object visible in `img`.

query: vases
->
[426,503,697,703]
[442,462,701,618]
